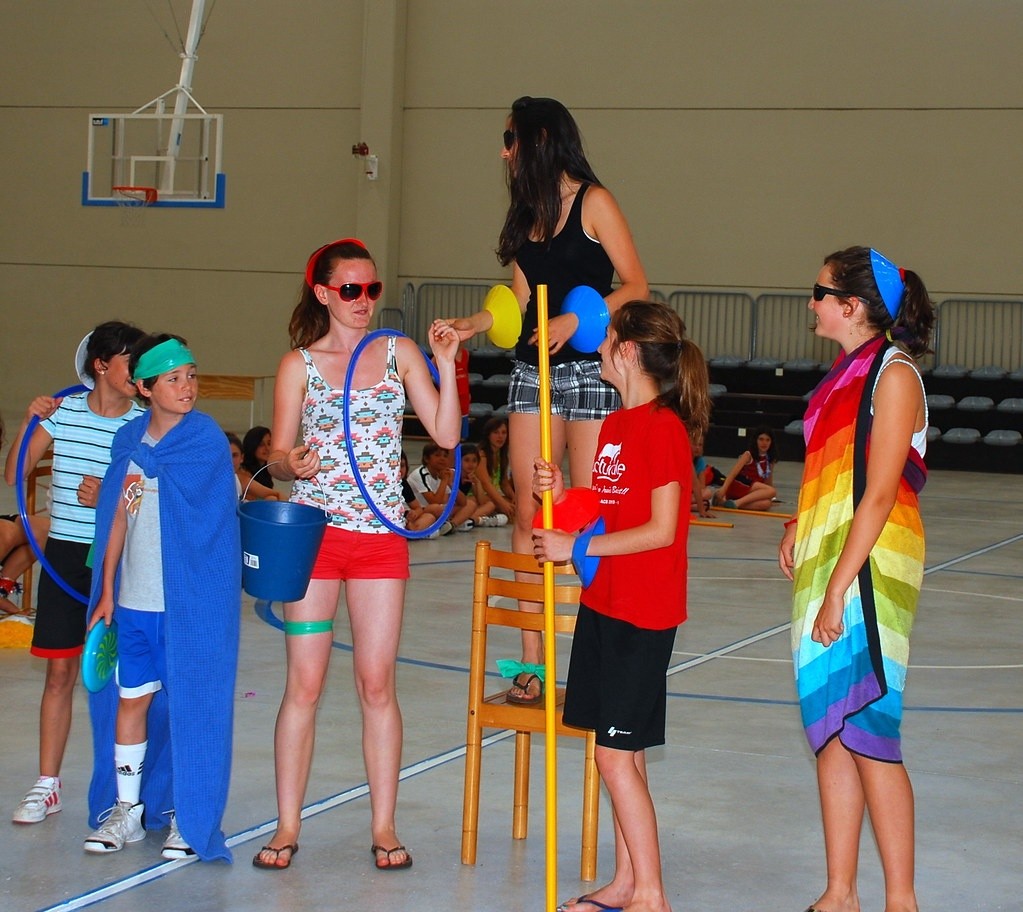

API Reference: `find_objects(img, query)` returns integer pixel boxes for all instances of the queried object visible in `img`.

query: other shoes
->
[702,500,710,511]
[439,521,453,535]
[712,491,725,506]
[424,529,439,540]
[804,905,822,912]
[456,517,475,532]
[482,514,508,527]
[725,499,738,508]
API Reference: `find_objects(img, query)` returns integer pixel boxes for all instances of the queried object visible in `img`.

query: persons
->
[782,247,936,912]
[400,417,515,540]
[84,336,240,864]
[446,94,651,701]
[6,321,146,822]
[529,300,708,912]
[0,514,50,620]
[224,426,288,502]
[690,427,779,518]
[253,240,460,869]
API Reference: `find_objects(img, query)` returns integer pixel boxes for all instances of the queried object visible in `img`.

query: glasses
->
[503,129,521,150]
[814,283,870,304]
[320,281,383,302]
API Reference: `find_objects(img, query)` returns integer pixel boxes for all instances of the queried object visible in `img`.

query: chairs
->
[460,541,602,887]
[22,441,54,611]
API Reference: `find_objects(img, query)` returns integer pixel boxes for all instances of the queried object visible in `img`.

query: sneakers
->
[12,777,63,823]
[160,808,198,858]
[83,800,147,853]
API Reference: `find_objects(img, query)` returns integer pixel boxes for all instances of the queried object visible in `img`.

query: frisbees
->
[79,616,119,695]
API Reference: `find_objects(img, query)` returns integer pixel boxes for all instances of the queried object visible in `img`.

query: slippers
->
[371,843,412,869]
[252,842,298,869]
[557,894,623,912]
[496,659,546,704]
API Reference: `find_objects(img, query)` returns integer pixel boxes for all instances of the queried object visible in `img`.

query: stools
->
[404,344,1023,470]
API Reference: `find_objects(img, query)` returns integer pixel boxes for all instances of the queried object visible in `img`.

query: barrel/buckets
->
[236,460,333,604]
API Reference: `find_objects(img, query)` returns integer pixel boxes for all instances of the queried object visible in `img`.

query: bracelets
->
[784,517,797,528]
